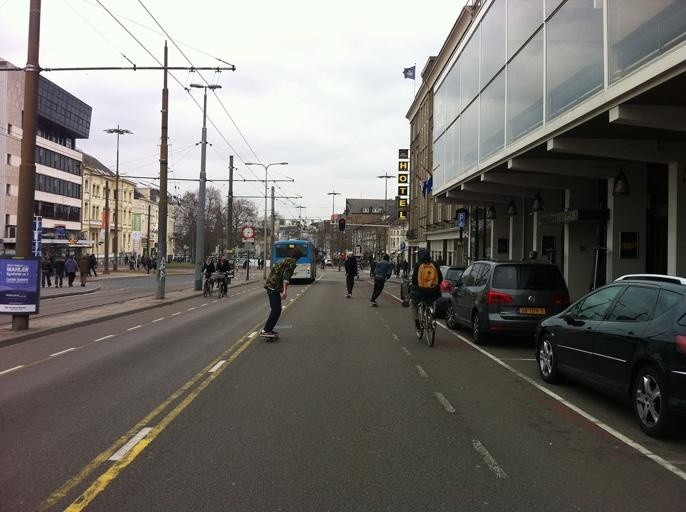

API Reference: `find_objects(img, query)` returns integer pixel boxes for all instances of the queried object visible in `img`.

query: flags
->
[403,67,415,80]
[420,180,427,199]
[426,176,433,194]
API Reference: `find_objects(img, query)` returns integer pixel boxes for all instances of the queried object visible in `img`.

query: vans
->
[446,259,571,343]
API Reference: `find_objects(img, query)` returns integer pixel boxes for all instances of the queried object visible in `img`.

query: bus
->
[274,238,317,283]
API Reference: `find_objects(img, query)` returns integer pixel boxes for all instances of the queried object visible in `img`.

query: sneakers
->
[260,327,279,335]
[369,299,377,304]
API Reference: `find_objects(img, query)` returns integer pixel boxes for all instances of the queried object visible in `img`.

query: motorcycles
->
[320,257,325,270]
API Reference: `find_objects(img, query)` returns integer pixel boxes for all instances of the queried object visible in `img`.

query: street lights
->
[244,161,288,279]
[294,203,307,241]
[105,124,132,272]
[190,84,223,291]
[327,192,341,267]
[376,172,396,260]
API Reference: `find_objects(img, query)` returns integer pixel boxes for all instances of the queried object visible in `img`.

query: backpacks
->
[417,262,439,290]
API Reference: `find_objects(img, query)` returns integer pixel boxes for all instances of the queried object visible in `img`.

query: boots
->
[414,319,422,331]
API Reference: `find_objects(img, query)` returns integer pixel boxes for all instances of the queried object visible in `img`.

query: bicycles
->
[216,270,234,299]
[414,300,437,347]
[201,272,215,298]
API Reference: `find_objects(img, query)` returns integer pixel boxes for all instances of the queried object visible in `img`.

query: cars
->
[327,259,333,266]
[401,265,466,320]
[534,272,686,442]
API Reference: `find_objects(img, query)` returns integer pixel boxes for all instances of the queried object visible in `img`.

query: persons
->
[410,248,443,332]
[528,250,538,259]
[262,249,304,335]
[343,250,358,297]
[367,255,395,306]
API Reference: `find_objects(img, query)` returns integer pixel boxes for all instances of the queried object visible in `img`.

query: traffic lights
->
[338,218,346,231]
